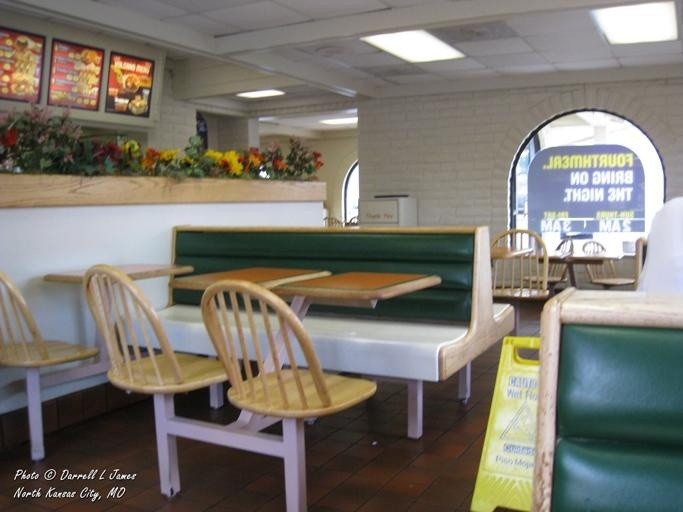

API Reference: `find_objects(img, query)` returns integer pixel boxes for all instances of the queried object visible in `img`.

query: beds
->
[488,245,536,260]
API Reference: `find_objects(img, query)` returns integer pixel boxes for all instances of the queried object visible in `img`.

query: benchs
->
[475,224,518,354]
[532,285,683,511]
[118,220,483,441]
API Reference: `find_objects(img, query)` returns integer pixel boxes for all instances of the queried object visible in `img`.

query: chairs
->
[80,258,240,498]
[196,268,381,512]
[488,225,550,302]
[0,272,99,463]
[523,233,573,285]
[582,238,635,289]
